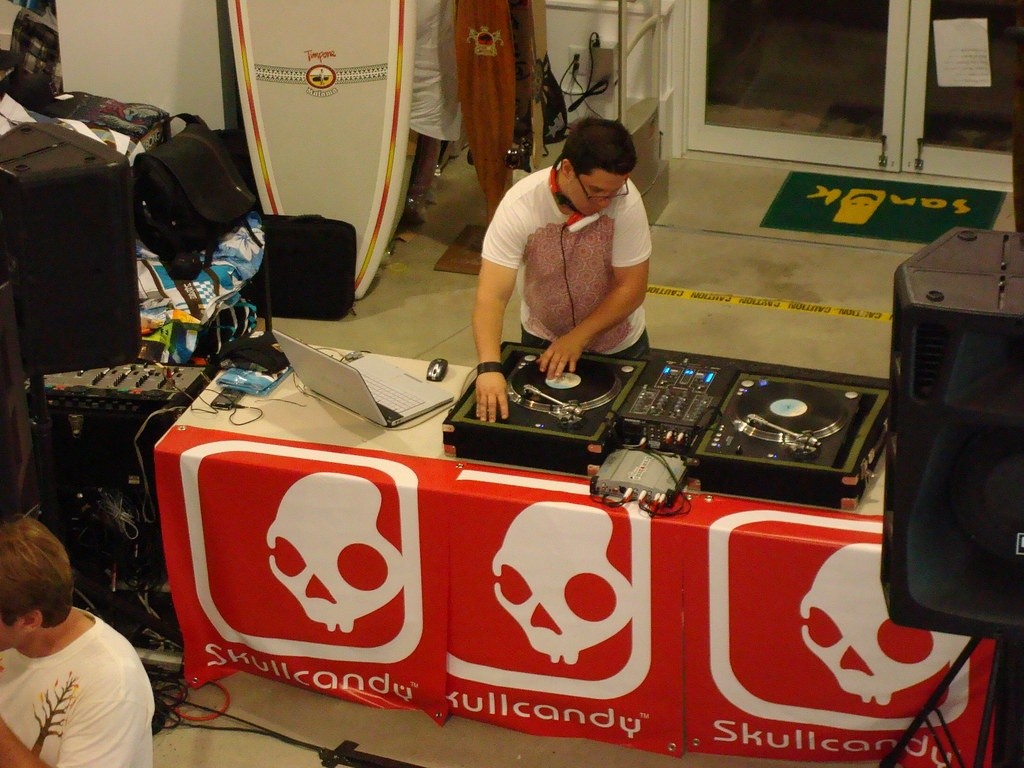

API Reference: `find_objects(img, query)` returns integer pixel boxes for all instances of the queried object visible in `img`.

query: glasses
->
[573,165,629,199]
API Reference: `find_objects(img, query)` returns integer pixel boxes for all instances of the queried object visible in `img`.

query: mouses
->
[427,358,448,382]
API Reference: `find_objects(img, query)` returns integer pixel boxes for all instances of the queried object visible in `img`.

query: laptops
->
[272,329,454,427]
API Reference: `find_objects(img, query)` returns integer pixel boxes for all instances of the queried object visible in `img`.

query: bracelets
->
[476,361,504,375]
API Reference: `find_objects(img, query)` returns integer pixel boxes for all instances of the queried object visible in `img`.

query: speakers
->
[876,226,1024,644]
[0,121,143,375]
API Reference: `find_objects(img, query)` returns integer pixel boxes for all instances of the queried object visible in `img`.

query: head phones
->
[549,153,601,233]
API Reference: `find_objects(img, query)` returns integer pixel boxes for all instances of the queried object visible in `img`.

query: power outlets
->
[568,45,588,75]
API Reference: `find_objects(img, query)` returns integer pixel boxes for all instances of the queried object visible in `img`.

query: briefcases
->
[258,214,358,320]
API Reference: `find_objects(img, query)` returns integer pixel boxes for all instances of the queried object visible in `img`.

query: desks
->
[155,332,996,768]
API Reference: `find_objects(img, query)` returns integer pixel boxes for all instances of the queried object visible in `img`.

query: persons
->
[403,1,461,223]
[475,118,652,424]
[0,513,157,768]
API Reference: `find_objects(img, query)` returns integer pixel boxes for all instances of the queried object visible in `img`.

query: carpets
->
[758,171,1007,246]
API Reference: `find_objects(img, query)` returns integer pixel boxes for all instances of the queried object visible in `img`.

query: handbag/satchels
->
[133,113,255,280]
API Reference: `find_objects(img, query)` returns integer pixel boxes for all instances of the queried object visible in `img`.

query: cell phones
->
[210,388,245,411]
